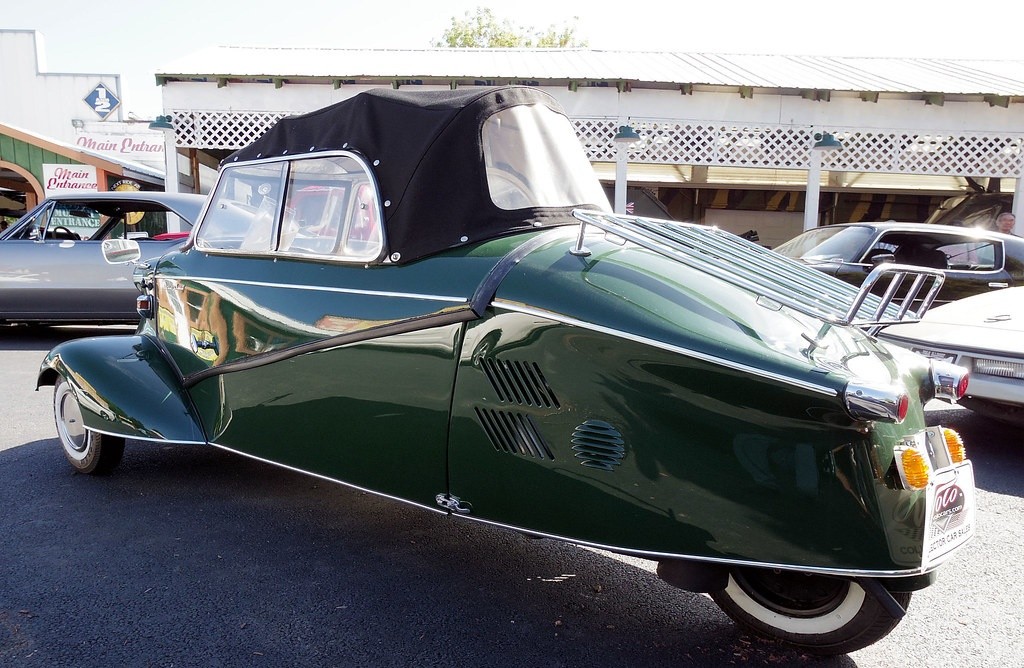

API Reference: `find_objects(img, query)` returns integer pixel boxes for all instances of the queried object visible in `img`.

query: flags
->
[626,201,635,215]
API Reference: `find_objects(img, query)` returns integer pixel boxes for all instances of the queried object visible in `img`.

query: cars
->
[859,286,1024,423]
[32,84,974,655]
[0,180,384,339]
[768,218,1024,315]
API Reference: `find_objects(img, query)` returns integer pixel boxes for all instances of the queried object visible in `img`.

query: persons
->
[0,221,8,230]
[979,212,1023,264]
